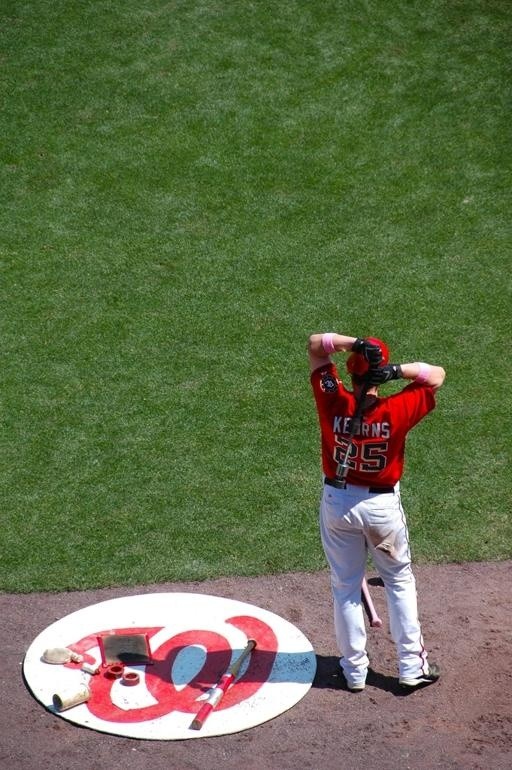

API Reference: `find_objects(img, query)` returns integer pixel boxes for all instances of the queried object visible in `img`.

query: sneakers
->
[399,660,441,690]
[327,669,365,690]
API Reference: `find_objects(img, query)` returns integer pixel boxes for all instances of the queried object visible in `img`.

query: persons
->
[307,329,445,691]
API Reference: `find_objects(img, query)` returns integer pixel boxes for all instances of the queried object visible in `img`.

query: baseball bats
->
[360,578,383,628]
[193,638,256,730]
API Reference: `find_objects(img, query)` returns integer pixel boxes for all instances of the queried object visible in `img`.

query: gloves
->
[363,363,404,387]
[352,338,382,366]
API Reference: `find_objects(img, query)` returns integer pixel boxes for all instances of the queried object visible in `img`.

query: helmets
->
[346,336,389,376]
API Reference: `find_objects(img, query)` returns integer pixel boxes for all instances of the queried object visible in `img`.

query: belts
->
[323,477,394,494]
